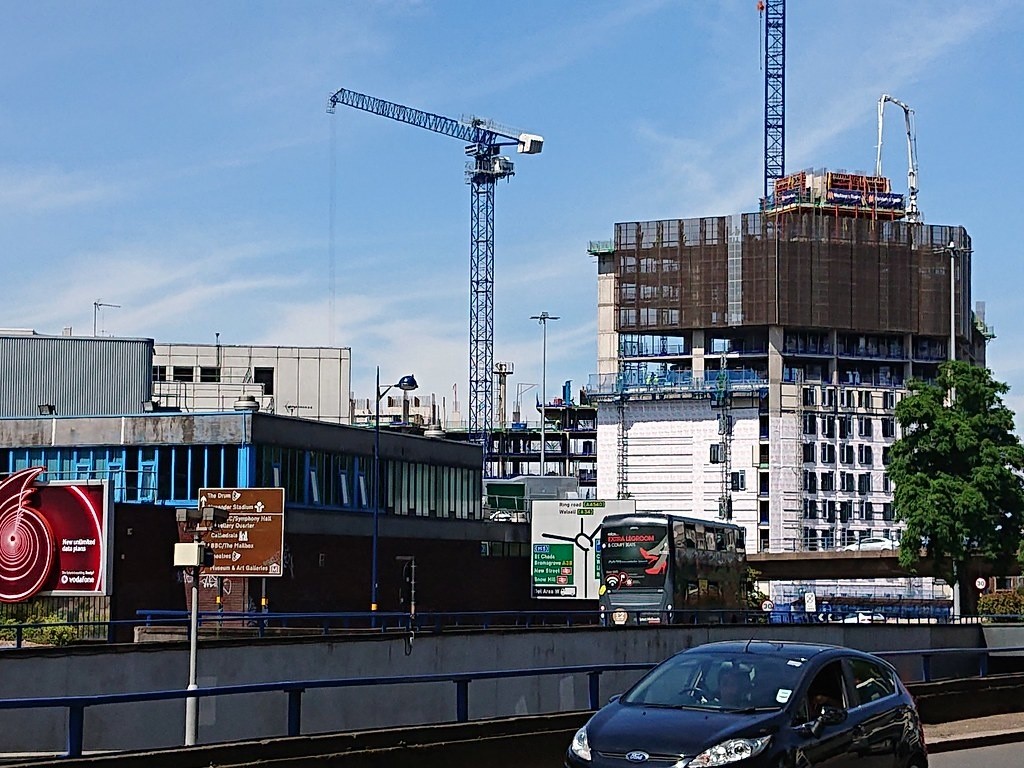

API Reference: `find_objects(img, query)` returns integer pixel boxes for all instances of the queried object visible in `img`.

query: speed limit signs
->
[975,578,986,589]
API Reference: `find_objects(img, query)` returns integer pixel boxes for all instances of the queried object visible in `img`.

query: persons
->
[717,664,749,703]
[646,375,659,392]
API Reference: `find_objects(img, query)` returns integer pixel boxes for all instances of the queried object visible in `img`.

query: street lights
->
[368,363,419,624]
[173,505,233,748]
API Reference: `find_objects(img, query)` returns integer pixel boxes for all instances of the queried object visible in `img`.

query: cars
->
[559,639,932,768]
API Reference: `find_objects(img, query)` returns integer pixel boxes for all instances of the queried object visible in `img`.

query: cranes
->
[325,87,544,475]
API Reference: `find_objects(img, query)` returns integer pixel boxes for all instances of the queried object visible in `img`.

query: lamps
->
[38,404,58,415]
[141,400,159,413]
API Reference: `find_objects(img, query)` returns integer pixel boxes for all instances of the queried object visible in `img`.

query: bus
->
[598,511,750,626]
